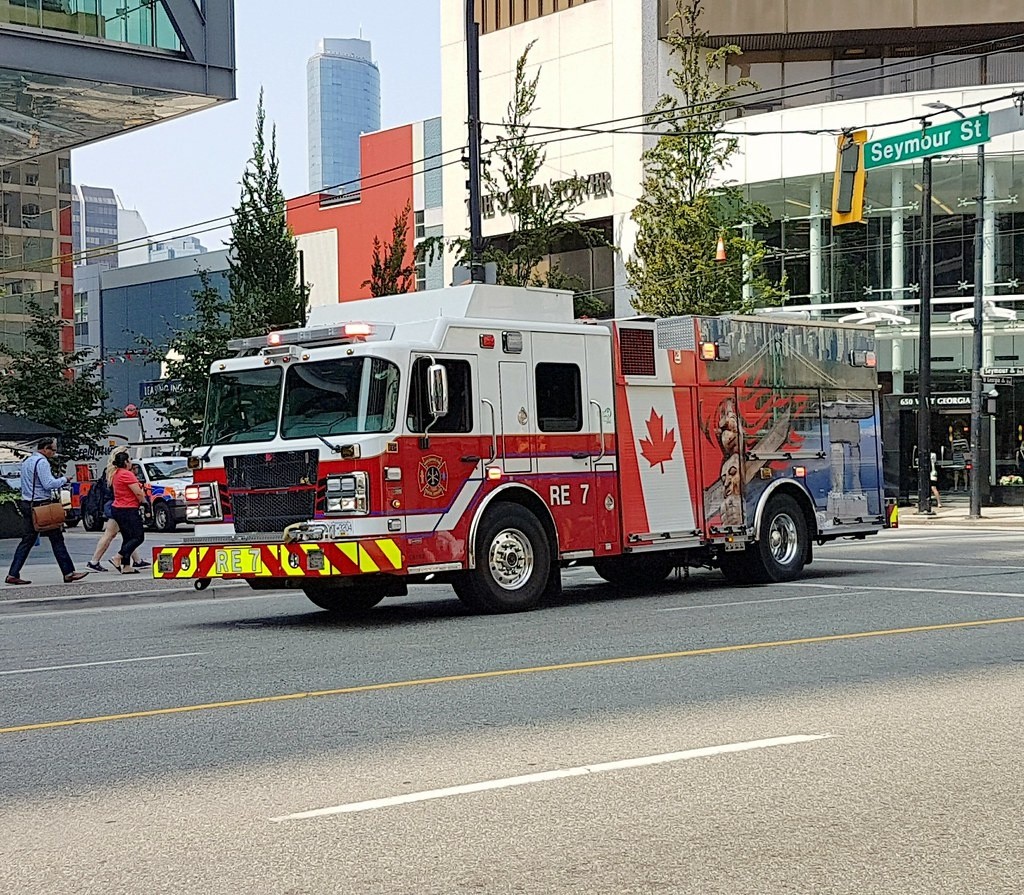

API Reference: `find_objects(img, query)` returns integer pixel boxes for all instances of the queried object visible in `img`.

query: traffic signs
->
[862,112,989,170]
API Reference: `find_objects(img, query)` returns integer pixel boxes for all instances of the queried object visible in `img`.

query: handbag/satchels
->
[32,501,65,530]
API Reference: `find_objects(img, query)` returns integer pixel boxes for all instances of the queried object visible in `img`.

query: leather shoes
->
[5,576,32,584]
[64,571,89,583]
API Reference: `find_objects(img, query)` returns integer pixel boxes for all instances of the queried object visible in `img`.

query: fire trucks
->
[151,282,900,615]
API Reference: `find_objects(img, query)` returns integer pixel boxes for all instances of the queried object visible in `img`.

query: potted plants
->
[989,474,1024,507]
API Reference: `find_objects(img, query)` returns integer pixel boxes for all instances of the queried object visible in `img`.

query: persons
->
[4,436,89,584]
[86,446,151,573]
[108,452,145,574]
[929,451,942,508]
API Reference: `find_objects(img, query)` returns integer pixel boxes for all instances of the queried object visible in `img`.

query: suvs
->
[0,460,81,527]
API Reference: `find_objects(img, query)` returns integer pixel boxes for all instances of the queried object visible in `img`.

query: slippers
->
[108,559,121,573]
[122,569,140,574]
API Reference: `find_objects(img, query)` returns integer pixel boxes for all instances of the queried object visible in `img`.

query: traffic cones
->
[712,237,727,261]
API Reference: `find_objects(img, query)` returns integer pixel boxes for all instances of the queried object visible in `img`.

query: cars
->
[943,415,971,450]
[81,456,194,532]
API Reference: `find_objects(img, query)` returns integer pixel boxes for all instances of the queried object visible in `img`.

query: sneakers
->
[133,559,152,569]
[86,561,108,573]
